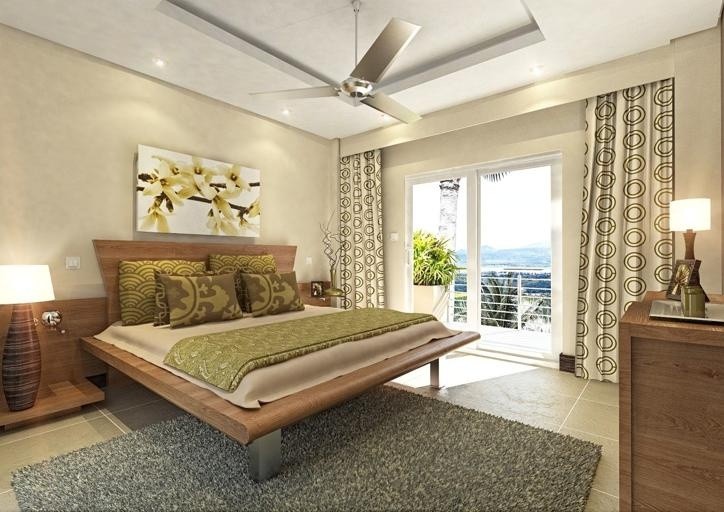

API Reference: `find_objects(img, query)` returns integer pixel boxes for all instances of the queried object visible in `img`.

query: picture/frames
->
[311,280,324,298]
[666,260,709,299]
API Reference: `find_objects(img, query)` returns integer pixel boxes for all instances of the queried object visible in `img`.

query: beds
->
[79,240,481,482]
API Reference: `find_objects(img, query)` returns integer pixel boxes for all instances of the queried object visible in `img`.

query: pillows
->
[118,254,305,329]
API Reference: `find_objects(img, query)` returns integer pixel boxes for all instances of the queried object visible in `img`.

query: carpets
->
[11,384,601,512]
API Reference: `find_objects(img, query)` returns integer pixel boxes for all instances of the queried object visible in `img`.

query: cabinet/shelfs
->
[619,291,723,511]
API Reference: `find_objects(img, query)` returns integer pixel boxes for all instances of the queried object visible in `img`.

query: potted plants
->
[414,231,459,326]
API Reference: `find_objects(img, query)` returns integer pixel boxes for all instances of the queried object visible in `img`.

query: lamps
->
[0,263,56,412]
[670,199,712,259]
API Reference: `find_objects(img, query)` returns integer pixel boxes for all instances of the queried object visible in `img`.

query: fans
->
[248,0,423,128]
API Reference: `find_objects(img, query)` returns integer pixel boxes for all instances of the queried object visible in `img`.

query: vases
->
[323,268,343,298]
